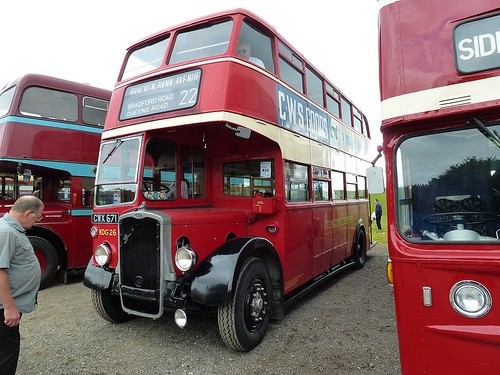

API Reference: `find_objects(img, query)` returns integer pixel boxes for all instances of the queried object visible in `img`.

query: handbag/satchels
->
[371,211,376,220]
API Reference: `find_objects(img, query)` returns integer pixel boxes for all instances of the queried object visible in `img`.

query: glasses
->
[31,211,42,221]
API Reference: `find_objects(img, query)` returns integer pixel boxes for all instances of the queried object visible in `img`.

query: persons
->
[1,194,44,375]
[374,198,383,230]
[264,189,273,197]
[238,42,266,71]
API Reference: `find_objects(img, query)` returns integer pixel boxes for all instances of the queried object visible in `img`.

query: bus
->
[377,0,500,375]
[0,73,113,288]
[81,8,379,351]
